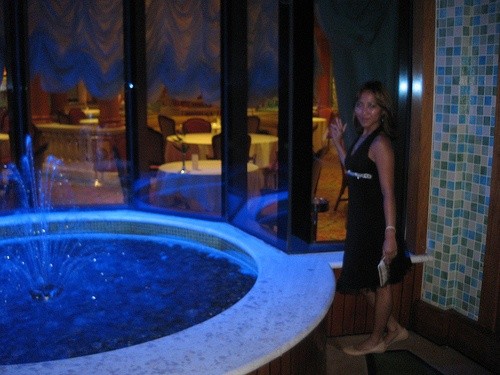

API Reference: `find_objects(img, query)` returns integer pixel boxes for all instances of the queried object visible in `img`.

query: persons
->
[329,81,410,357]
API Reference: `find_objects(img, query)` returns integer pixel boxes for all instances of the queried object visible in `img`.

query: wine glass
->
[174,144,190,174]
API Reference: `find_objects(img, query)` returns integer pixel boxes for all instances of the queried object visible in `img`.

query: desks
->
[165,131,279,174]
[313,117,329,153]
[154,160,265,212]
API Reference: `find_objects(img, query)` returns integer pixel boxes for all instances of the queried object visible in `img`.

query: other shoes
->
[343,340,385,356]
[384,328,408,349]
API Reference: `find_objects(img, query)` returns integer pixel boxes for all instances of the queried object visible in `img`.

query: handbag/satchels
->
[378,226,397,287]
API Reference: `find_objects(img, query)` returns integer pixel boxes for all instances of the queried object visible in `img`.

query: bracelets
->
[385,226,396,234]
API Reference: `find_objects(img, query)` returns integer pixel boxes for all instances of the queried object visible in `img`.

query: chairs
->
[146,116,350,213]
